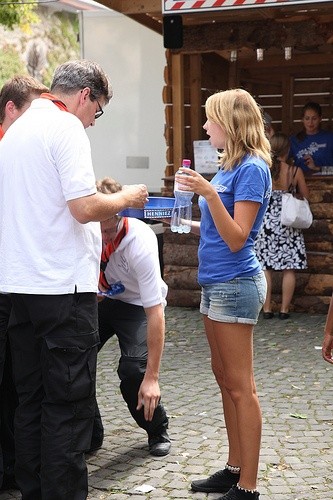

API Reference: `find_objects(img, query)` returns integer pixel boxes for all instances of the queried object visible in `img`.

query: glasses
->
[80,88,103,119]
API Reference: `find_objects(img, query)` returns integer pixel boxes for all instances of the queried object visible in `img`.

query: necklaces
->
[40,93,68,112]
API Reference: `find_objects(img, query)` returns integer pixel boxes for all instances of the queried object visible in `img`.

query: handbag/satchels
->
[280,166,313,228]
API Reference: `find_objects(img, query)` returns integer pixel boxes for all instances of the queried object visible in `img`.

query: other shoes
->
[216,484,260,500]
[192,469,241,492]
[279,312,289,319]
[264,312,273,319]
[148,430,171,455]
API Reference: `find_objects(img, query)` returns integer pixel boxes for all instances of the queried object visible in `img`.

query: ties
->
[98,218,128,292]
[39,91,70,114]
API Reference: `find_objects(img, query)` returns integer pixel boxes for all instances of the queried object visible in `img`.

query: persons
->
[254,134,310,321]
[289,102,333,174]
[0,59,149,500]
[91,177,171,457]
[174,88,273,500]
[0,74,50,141]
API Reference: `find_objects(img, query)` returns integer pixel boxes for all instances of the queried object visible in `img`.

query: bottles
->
[170,159,194,233]
